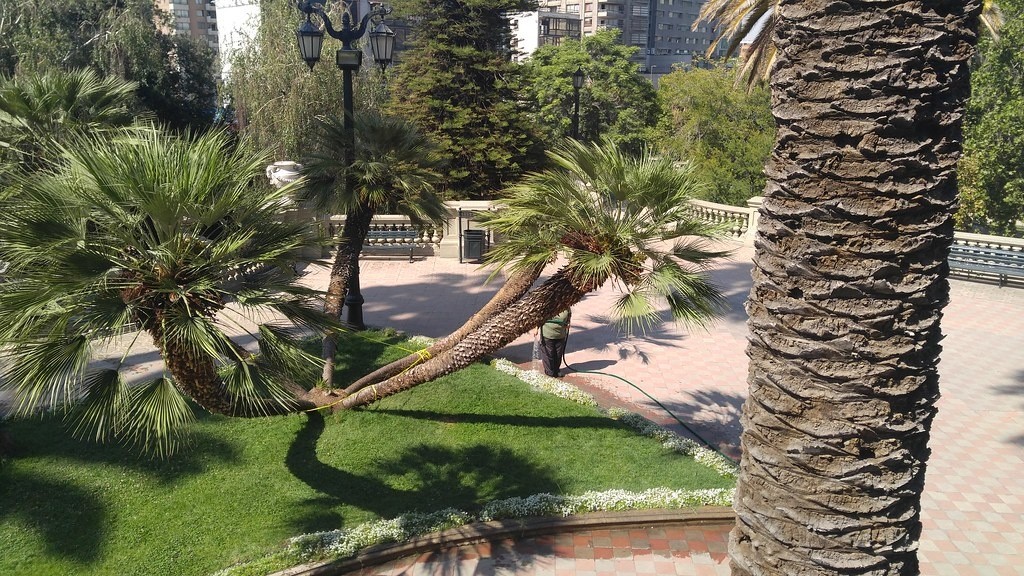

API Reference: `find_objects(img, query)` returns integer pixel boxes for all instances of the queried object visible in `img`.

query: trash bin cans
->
[463,228,486,261]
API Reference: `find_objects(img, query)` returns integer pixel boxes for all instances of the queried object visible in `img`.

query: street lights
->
[571,64,584,141]
[296,0,396,328]
[650,65,657,82]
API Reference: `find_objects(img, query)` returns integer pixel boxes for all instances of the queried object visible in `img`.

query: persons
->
[536,305,571,378]
